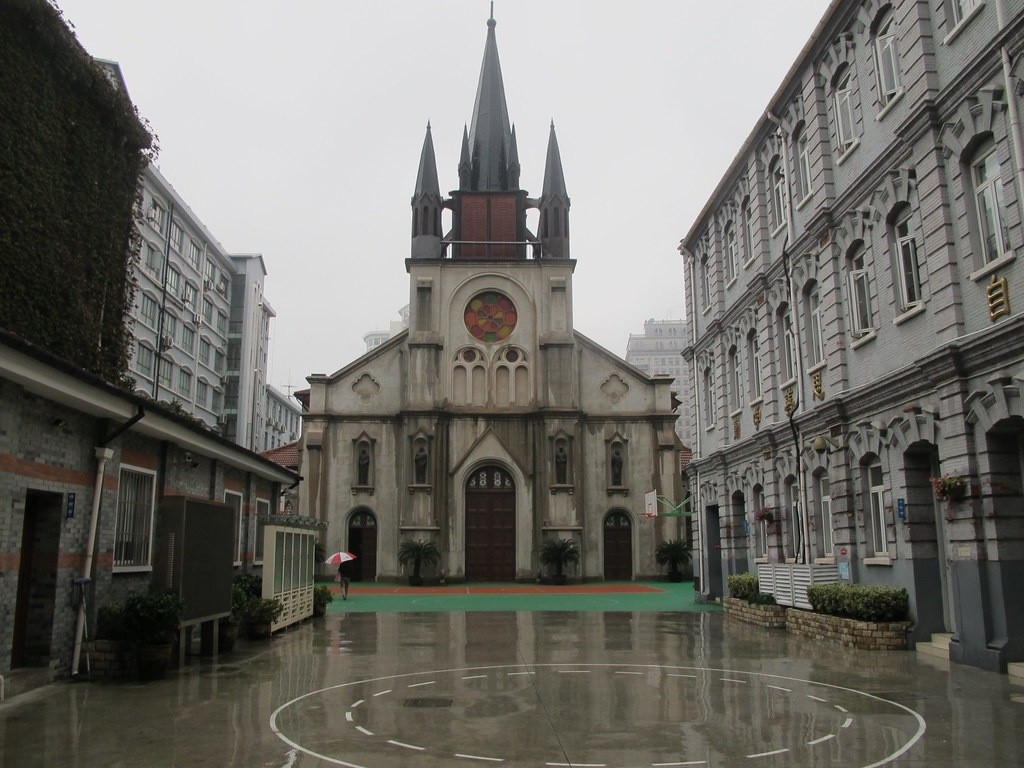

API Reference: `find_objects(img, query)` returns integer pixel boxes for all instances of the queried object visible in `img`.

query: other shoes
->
[342,594,347,600]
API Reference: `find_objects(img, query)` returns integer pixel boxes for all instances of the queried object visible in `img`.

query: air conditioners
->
[148,209,156,219]
[182,292,191,300]
[221,376,229,384]
[194,314,201,323]
[218,414,227,424]
[206,280,214,289]
[164,336,173,348]
[282,425,286,433]
[275,422,281,430]
[268,418,273,426]
[218,282,225,291]
[292,433,296,439]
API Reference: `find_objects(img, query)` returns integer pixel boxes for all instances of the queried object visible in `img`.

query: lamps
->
[54,419,66,427]
[192,463,200,469]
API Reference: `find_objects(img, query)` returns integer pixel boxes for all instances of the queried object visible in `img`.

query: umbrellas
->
[325,552,356,564]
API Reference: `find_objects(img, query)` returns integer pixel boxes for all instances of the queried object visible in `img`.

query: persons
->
[338,561,351,601]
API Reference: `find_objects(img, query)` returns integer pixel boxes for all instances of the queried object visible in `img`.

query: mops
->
[71,577,93,682]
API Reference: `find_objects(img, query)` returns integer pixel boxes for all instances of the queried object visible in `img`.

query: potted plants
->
[98,587,187,682]
[398,539,441,586]
[313,586,333,617]
[540,539,580,585]
[200,584,245,652]
[241,597,284,640]
[658,539,693,582]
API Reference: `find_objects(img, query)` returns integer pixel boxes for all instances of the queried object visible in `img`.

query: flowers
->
[755,507,772,523]
[930,474,968,501]
